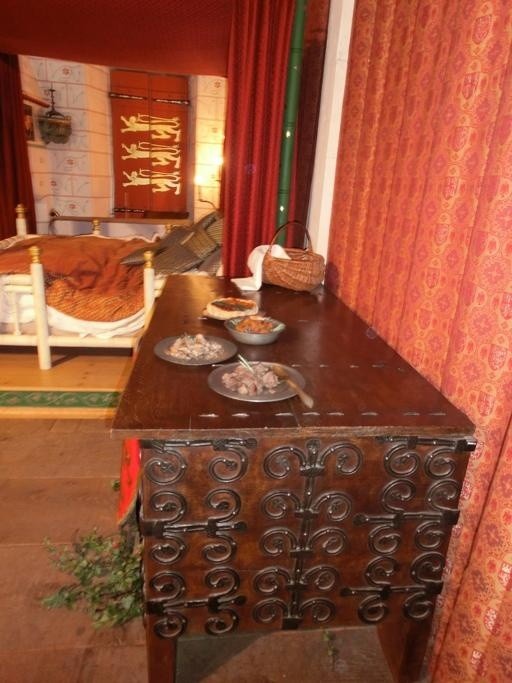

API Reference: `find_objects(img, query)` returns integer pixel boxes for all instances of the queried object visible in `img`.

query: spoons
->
[274,365,314,410]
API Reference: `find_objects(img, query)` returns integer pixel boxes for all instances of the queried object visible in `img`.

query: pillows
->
[120,210,224,279]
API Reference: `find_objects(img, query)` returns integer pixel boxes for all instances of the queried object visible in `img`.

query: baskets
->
[252,221,325,291]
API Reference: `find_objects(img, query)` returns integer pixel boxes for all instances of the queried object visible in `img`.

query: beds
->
[1,202,223,369]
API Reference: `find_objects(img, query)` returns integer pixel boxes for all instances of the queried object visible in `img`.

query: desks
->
[108,273,478,682]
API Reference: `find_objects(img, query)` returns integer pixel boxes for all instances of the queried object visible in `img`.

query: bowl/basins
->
[225,316,286,345]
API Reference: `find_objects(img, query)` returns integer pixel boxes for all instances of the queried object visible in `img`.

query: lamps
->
[36,87,72,142]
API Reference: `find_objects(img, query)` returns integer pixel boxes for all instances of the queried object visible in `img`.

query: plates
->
[208,360,305,403]
[154,334,238,365]
[200,307,227,322]
